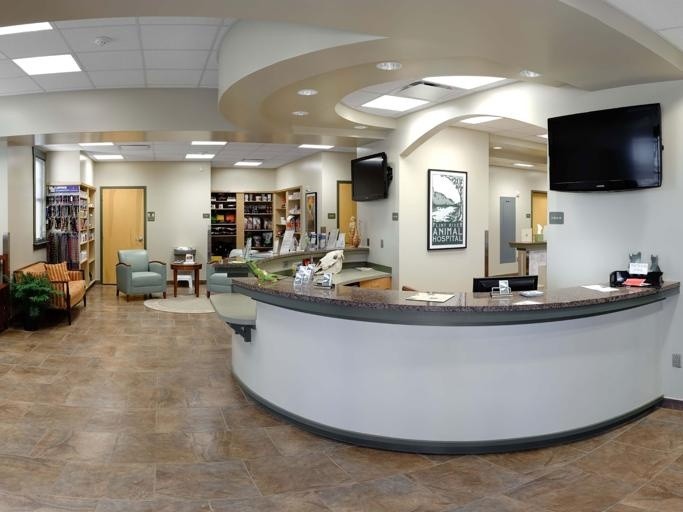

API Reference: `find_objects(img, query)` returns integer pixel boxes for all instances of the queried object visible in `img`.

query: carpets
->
[144,294,215,313]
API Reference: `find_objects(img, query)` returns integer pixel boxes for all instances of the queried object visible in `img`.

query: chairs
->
[13,261,87,325]
[206,249,258,298]
[116,248,167,302]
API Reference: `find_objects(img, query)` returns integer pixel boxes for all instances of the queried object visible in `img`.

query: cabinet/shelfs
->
[211,191,236,258]
[45,183,96,291]
[273,186,303,250]
[244,192,273,252]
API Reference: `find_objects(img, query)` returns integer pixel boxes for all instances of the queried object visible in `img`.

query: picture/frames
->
[427,169,468,251]
[304,192,318,245]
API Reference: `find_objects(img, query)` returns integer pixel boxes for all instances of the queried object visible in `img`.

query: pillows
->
[44,261,71,283]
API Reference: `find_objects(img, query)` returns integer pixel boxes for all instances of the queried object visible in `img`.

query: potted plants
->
[10,273,63,330]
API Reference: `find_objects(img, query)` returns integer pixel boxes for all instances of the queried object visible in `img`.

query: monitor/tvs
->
[351,152,392,202]
[545,100,665,194]
